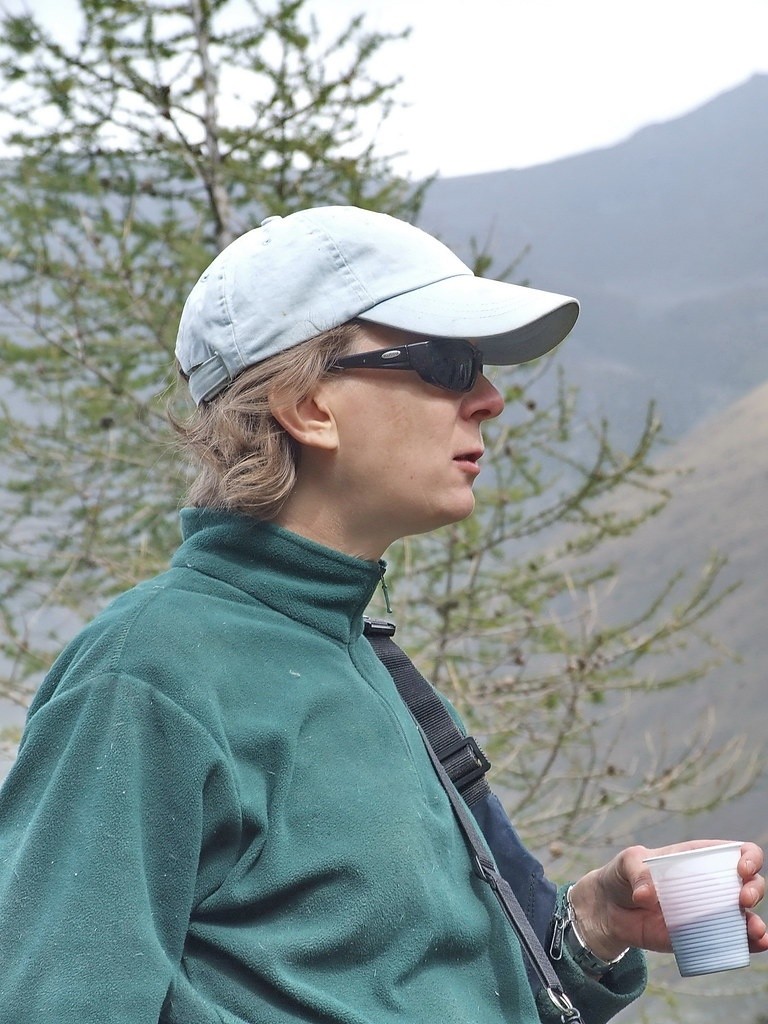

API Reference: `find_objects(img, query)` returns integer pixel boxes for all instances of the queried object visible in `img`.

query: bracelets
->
[557,879,630,974]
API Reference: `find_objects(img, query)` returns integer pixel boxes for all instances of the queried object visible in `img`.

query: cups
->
[642,842,751,977]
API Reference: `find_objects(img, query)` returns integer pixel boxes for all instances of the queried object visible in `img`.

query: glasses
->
[327,337,485,392]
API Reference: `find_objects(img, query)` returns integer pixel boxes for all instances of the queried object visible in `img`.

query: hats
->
[172,205,579,407]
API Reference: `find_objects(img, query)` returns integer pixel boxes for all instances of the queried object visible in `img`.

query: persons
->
[0,206,767,1024]
